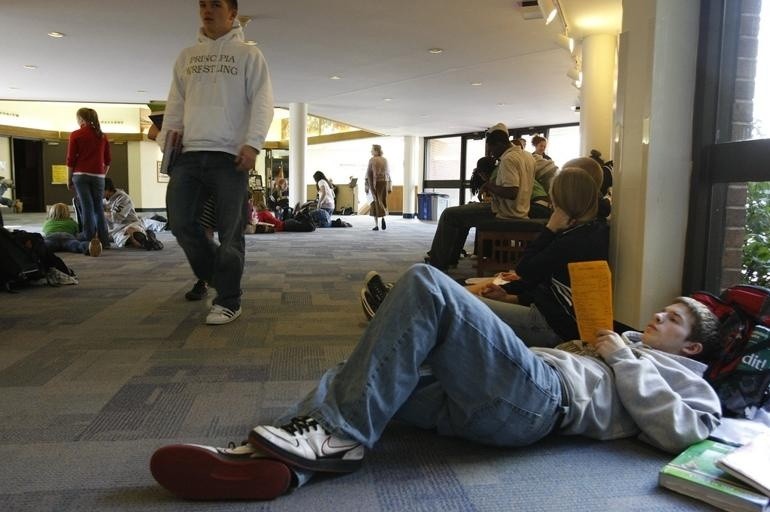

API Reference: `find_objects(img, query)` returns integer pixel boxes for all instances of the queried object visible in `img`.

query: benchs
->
[476,218,547,278]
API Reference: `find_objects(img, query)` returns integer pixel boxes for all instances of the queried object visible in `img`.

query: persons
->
[532,135,550,161]
[424,123,536,271]
[471,156,551,206]
[562,156,605,191]
[360,165,609,349]
[0,176,23,214]
[269,178,290,209]
[146,95,218,301]
[67,107,112,251]
[98,176,165,251]
[42,203,102,257]
[590,150,613,195]
[365,144,391,230]
[312,171,352,227]
[148,263,722,502]
[155,1,271,326]
[257,202,315,232]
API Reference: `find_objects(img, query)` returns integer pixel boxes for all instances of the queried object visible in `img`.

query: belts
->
[535,199,553,209]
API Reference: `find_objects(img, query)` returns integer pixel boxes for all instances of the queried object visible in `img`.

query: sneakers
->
[132,231,152,251]
[470,254,478,260]
[206,304,241,324]
[5,179,15,189]
[347,221,353,228]
[372,226,379,231]
[149,441,291,499]
[293,202,301,215]
[424,257,449,274]
[447,262,459,273]
[146,230,164,250]
[248,416,365,473]
[16,201,23,213]
[88,239,103,257]
[363,270,391,303]
[185,279,209,300]
[360,287,380,321]
[301,201,314,209]
[382,218,386,229]
[341,220,348,227]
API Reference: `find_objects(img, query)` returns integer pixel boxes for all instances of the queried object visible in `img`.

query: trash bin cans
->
[418,194,450,221]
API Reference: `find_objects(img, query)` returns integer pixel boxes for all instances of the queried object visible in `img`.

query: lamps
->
[567,62,582,79]
[554,25,574,53]
[539,0,557,24]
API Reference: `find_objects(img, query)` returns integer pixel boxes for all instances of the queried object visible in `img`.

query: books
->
[719,424,769,502]
[654,422,769,512]
[160,130,184,176]
[567,262,615,349]
[147,100,168,126]
